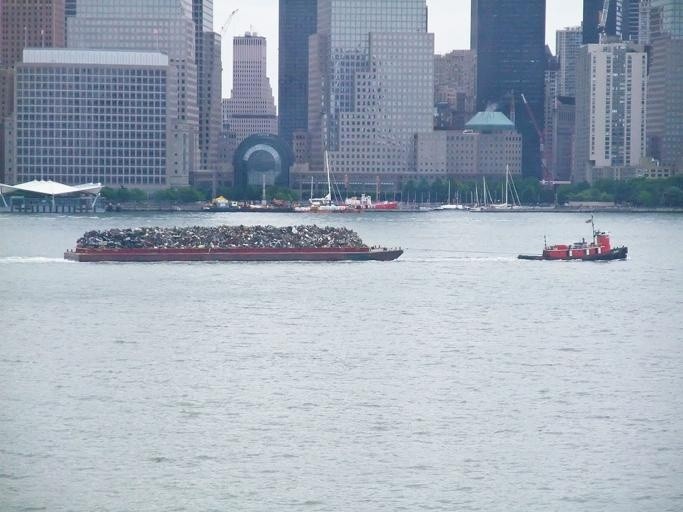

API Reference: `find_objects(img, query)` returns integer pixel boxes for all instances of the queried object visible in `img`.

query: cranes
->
[596,0,610,44]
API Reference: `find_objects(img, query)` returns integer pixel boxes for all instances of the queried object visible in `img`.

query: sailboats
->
[417,164,554,210]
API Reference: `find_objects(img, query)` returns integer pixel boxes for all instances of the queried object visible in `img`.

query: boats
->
[292,150,398,211]
[516,215,628,261]
[63,246,405,262]
